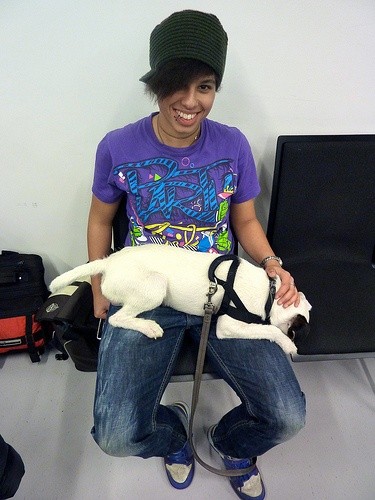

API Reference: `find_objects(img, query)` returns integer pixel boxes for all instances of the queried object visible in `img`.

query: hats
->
[139,10,228,90]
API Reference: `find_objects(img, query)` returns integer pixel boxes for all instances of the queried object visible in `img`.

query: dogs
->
[48,241,313,357]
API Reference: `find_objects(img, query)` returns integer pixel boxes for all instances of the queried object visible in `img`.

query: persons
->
[87,10,306,500]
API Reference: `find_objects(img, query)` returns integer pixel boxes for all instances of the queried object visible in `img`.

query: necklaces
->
[156,115,201,147]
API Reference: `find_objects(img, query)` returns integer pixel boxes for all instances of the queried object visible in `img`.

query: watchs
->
[260,256,283,267]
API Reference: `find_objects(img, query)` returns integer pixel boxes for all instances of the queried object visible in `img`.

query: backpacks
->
[0,250,49,362]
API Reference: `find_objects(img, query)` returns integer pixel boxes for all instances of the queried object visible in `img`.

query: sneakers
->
[164,401,194,489]
[207,424,265,500]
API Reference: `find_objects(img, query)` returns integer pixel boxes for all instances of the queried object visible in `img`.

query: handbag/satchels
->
[38,282,81,360]
